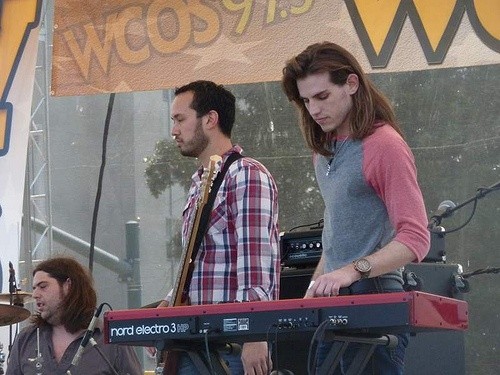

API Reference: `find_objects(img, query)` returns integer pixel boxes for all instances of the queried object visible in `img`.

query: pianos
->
[103,291,470,375]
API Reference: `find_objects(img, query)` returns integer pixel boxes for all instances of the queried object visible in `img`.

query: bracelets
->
[308,281,316,289]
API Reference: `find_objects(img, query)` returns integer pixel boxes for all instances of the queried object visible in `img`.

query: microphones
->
[9,262,16,293]
[429,200,456,229]
[72,303,103,366]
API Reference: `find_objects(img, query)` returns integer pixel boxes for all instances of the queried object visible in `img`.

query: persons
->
[281,41,430,375]
[5,257,145,375]
[147,81,280,375]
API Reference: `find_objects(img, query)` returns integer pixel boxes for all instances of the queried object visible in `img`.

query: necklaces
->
[325,135,350,176]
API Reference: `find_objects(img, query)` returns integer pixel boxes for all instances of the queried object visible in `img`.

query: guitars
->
[156,156,224,374]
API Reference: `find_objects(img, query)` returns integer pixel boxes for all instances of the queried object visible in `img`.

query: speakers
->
[272,263,468,375]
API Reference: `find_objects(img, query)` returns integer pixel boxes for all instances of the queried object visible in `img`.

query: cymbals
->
[0,303,30,326]
[0,290,33,302]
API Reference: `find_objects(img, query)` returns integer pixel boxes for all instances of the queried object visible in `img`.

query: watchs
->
[352,257,372,283]
